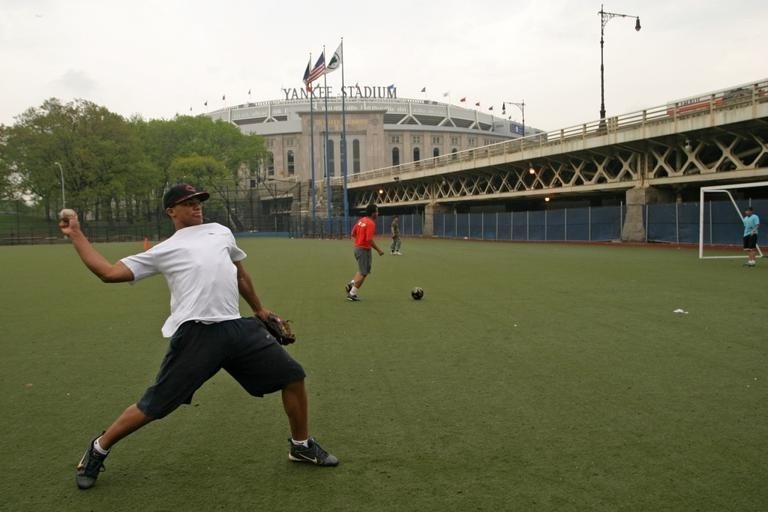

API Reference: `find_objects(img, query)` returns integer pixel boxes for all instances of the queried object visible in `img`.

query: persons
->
[344,203,385,302]
[58,183,340,490]
[742,207,760,266]
[389,215,403,256]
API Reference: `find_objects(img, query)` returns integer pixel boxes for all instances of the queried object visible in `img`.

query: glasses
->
[178,198,201,207]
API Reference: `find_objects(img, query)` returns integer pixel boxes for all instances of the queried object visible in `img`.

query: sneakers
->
[743,262,756,268]
[76,430,111,489]
[345,281,360,301]
[390,249,402,256]
[288,436,339,466]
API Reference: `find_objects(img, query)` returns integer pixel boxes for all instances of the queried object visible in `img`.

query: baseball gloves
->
[255,312,296,345]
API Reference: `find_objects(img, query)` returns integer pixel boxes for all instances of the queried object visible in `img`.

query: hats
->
[163,183,209,208]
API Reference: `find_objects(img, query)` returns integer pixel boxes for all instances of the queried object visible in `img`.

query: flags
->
[326,43,342,75]
[302,58,312,91]
[308,51,327,86]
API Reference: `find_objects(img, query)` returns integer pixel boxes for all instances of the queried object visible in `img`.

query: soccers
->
[412,287,423,299]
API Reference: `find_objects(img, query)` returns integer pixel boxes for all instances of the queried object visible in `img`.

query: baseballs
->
[60,209,76,222]
